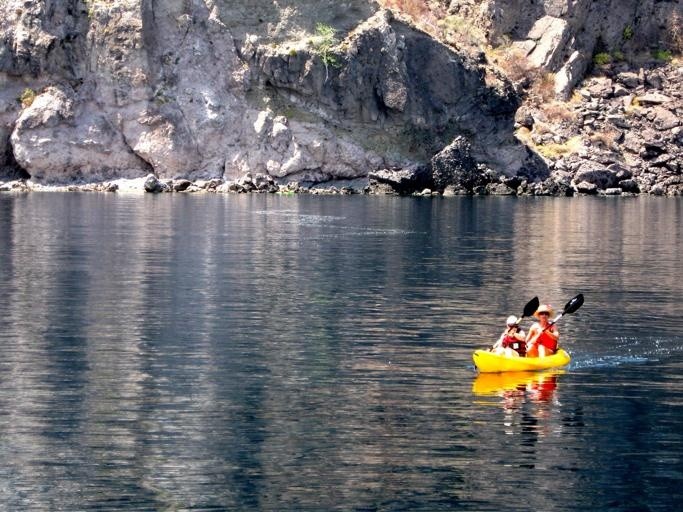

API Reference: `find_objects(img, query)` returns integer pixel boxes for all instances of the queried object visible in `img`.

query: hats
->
[533,305,555,319]
[506,315,518,328]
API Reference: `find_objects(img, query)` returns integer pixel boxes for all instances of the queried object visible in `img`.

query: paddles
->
[529,294,584,344]
[495,296,540,346]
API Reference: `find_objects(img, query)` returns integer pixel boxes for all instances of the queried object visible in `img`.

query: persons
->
[526,304,559,358]
[487,315,527,358]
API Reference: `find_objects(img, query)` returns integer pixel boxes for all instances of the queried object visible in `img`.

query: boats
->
[473,348,570,372]
[472,370,566,394]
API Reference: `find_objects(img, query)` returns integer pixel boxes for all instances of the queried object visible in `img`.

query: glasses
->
[539,312,549,315]
